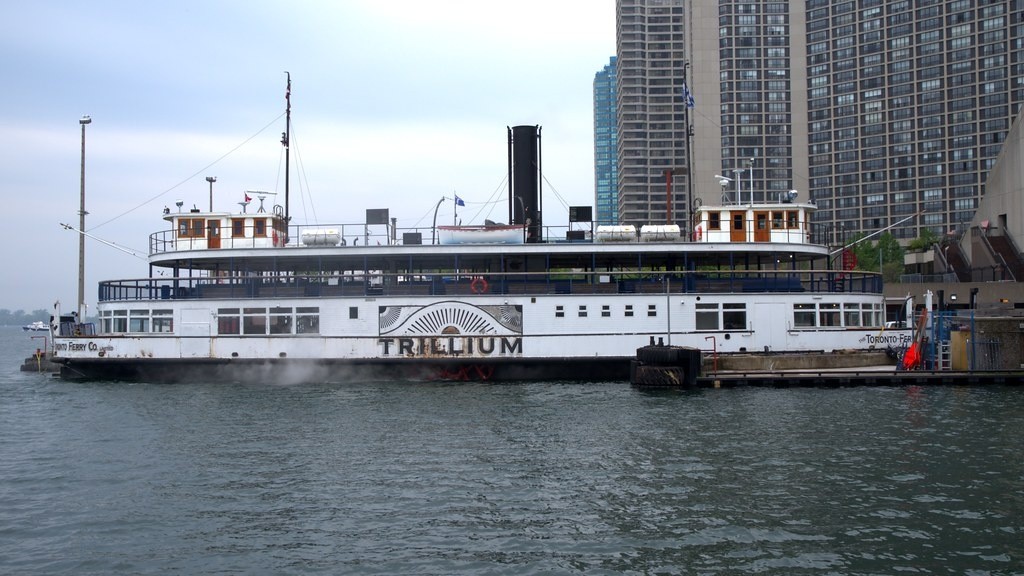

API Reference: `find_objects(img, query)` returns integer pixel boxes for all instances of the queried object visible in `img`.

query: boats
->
[24,321,51,331]
[49,72,935,382]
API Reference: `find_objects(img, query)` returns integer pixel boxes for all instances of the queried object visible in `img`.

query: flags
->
[682,83,695,107]
[246,194,252,202]
[455,194,465,207]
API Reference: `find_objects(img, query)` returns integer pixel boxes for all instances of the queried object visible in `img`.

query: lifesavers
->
[471,278,488,294]
[698,226,702,240]
[273,232,278,247]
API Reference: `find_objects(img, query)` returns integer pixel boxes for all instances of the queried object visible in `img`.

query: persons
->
[525,217,533,240]
